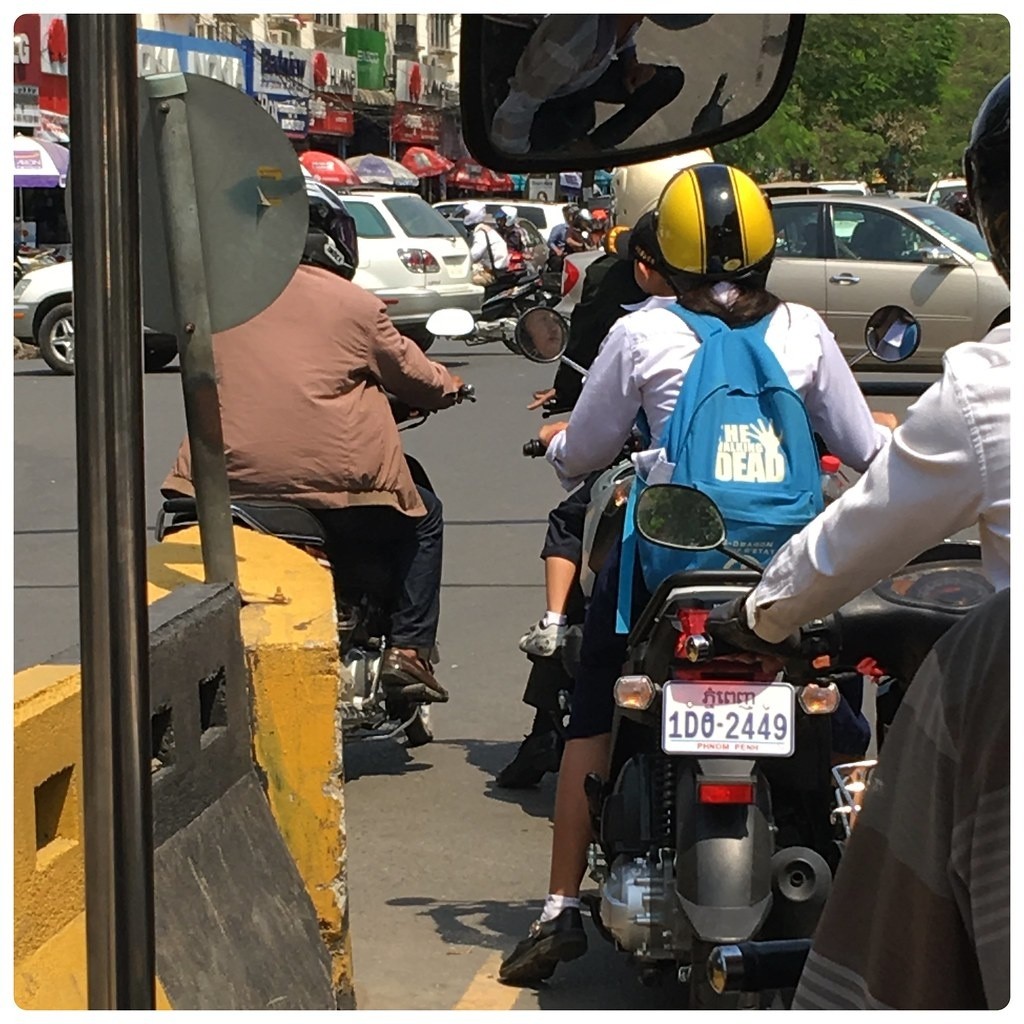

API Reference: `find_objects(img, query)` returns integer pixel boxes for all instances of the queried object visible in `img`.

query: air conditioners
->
[268,29,291,45]
[219,22,236,43]
[195,23,216,40]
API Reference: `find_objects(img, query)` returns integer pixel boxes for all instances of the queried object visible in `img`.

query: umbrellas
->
[448,154,513,203]
[344,152,420,192]
[400,146,455,204]
[14,133,70,244]
[296,150,361,191]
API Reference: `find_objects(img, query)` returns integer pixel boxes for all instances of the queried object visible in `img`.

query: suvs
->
[15,191,481,378]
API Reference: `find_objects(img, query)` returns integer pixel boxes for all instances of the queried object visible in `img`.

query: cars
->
[761,192,1010,396]
[13,177,1011,398]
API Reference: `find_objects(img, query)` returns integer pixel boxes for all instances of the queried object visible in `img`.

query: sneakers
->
[517,618,567,657]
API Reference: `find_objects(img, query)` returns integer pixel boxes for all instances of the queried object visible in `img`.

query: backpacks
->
[634,303,825,592]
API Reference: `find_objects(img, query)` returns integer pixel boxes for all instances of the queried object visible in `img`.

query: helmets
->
[562,203,580,223]
[654,162,776,282]
[495,206,517,228]
[961,71,1011,295]
[572,208,592,232]
[303,177,359,280]
[605,227,629,257]
[452,200,485,228]
[592,209,607,222]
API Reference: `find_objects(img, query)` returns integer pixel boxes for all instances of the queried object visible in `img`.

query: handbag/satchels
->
[492,268,516,281]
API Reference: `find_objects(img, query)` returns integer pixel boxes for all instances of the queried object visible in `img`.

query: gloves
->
[709,599,799,661]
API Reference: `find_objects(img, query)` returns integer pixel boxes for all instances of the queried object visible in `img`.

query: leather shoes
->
[381,648,450,702]
[495,723,567,789]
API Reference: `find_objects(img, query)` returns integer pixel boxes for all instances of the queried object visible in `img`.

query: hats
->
[629,208,663,271]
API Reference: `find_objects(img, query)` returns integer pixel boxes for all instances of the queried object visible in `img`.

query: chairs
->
[849,222,882,259]
[800,223,844,258]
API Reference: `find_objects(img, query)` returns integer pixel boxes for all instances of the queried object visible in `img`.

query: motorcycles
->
[154,381,480,770]
[511,303,898,1011]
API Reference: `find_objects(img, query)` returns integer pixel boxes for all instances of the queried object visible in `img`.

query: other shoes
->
[499,905,588,982]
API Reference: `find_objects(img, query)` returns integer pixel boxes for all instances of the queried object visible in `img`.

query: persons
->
[158,178,466,703]
[496,148,898,989]
[461,202,610,286]
[704,69,1010,1010]
